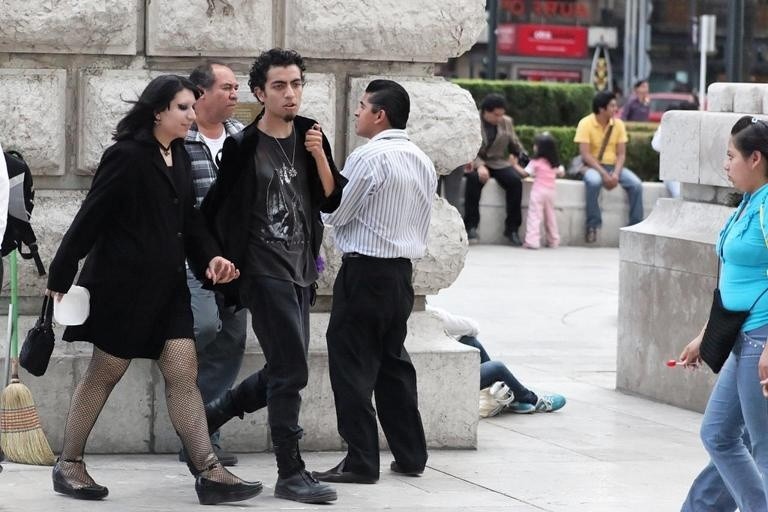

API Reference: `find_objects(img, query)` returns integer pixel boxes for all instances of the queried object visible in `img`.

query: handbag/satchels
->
[18,292,55,377]
[519,153,530,169]
[479,379,514,417]
[700,298,750,374]
[567,153,590,179]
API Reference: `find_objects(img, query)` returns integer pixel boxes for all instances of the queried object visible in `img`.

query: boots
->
[176,396,232,474]
[274,441,338,502]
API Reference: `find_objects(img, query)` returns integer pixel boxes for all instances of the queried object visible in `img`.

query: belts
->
[344,254,362,258]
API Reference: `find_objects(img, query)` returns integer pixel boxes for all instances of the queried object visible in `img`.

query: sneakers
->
[587,227,597,242]
[178,436,239,464]
[504,229,522,245]
[535,392,565,412]
[508,401,535,414]
[468,227,478,239]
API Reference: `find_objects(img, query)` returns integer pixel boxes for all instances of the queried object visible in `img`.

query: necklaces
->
[157,137,172,157]
[262,117,298,178]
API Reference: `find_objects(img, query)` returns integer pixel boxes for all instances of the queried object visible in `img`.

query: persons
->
[310,78,438,484]
[197,48,349,505]
[0,139,8,475]
[431,310,566,414]
[44,73,264,505]
[621,80,650,122]
[180,60,246,465]
[680,423,754,512]
[436,93,565,250]
[680,116,768,512]
[573,90,644,243]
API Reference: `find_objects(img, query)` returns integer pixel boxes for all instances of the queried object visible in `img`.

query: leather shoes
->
[195,467,261,504]
[313,454,378,483]
[53,458,108,500]
[388,458,421,477]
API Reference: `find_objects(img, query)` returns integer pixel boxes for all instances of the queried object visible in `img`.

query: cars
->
[613,90,700,123]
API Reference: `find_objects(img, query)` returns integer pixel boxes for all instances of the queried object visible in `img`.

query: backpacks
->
[2,149,36,261]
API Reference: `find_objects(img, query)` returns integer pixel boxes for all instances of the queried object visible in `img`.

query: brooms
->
[1,248,58,466]
